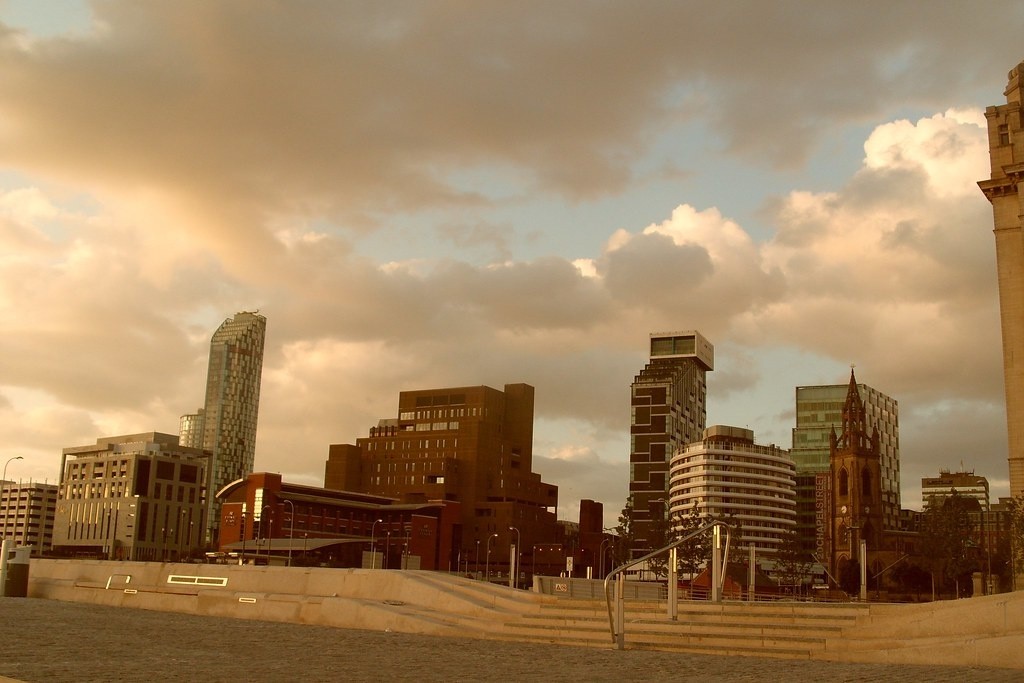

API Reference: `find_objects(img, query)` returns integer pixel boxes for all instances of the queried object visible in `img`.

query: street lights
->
[242,514,247,565]
[476,541,480,580]
[370,520,383,569]
[978,479,993,596]
[0,457,24,513]
[599,539,614,579]
[658,497,671,542]
[162,528,173,562]
[402,542,411,570]
[190,521,194,563]
[283,500,295,566]
[387,532,390,569]
[256,505,270,554]
[179,510,187,562]
[486,534,498,582]
[509,527,520,588]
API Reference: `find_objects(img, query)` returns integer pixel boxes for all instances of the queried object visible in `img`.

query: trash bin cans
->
[4,545,33,597]
[986,574,1000,595]
